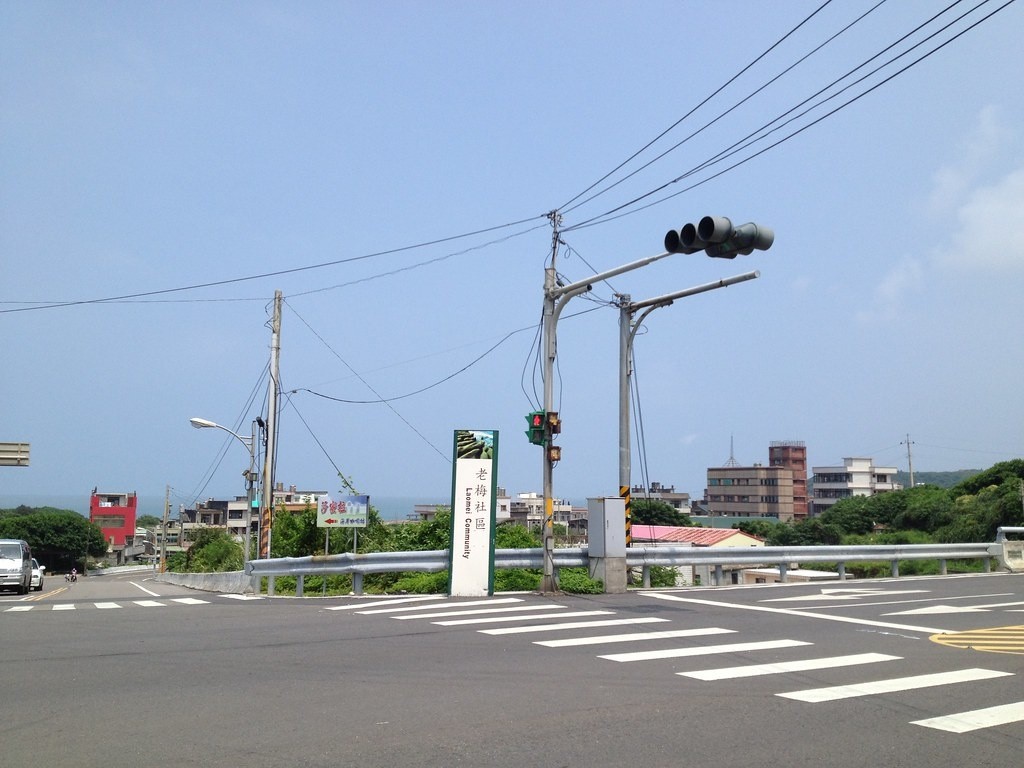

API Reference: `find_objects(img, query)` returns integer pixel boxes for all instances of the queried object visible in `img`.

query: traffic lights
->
[528,413,546,444]
[547,446,560,461]
[704,222,775,260]
[663,216,734,255]
[547,411,558,426]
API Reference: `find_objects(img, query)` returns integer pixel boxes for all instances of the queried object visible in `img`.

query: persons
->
[64,568,78,582]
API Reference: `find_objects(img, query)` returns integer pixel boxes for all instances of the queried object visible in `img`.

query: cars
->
[29,557,47,591]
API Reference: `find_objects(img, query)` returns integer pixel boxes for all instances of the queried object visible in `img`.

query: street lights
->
[190,417,256,562]
[137,527,158,569]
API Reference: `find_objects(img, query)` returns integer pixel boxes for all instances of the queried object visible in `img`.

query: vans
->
[0,538,33,595]
